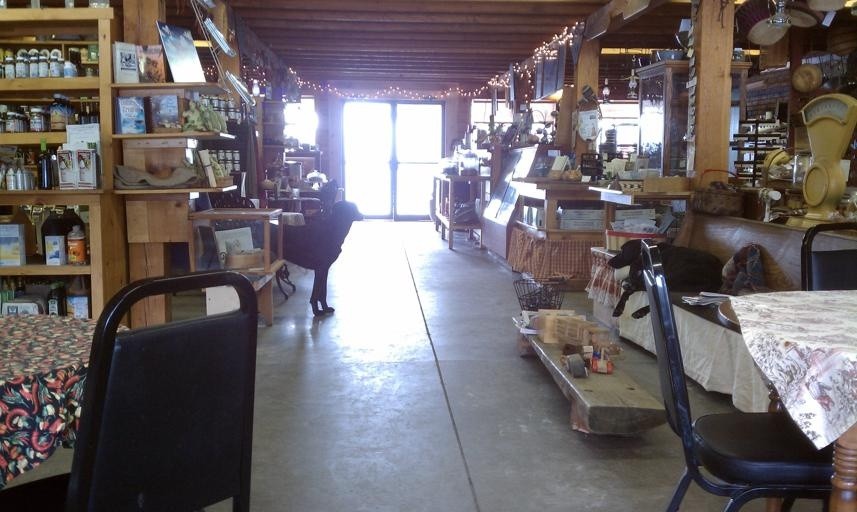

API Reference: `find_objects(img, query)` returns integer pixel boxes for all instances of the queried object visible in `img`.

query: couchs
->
[619,210,856,413]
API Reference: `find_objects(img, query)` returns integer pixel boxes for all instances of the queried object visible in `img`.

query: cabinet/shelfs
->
[433,172,493,250]
[510,176,693,292]
[631,56,846,187]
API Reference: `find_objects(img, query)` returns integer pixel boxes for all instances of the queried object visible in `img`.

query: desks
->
[0,314,131,487]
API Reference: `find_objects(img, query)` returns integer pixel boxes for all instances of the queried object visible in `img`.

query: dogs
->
[608,239,724,319]
[255,201,365,317]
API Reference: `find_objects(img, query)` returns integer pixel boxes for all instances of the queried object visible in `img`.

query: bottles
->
[188,95,244,174]
[0,47,105,321]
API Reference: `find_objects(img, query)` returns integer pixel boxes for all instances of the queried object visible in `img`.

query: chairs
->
[0,269,259,512]
